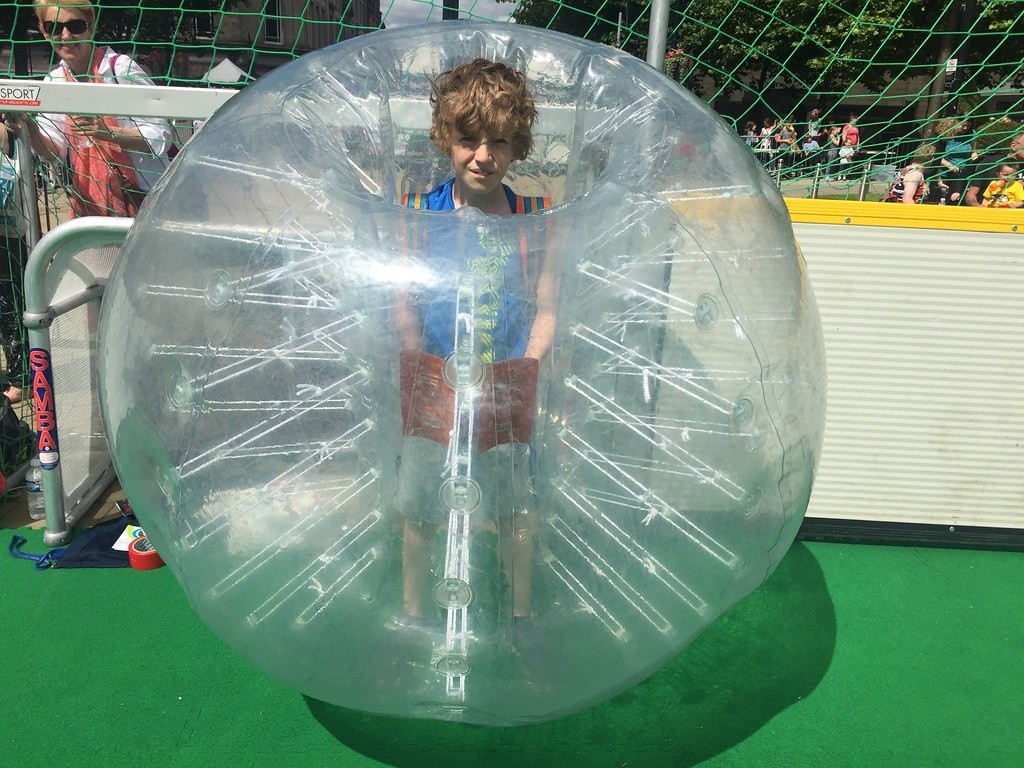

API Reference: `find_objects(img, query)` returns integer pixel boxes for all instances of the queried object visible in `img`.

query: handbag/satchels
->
[1,153,26,238]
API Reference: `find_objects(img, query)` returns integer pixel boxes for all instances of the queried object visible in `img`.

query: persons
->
[770,113,922,176]
[841,112,861,180]
[0,110,35,404]
[928,116,1024,210]
[802,108,829,177]
[1,0,178,286]
[781,115,798,179]
[824,127,844,182]
[837,138,855,181]
[759,118,778,176]
[742,121,760,159]
[367,58,560,654]
[877,142,938,205]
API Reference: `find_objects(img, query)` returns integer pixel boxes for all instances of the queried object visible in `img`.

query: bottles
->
[25,459,45,520]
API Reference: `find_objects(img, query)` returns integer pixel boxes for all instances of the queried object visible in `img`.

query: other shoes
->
[378,613,431,689]
[508,616,555,691]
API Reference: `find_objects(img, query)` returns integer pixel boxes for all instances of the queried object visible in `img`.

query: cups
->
[70,115,94,148]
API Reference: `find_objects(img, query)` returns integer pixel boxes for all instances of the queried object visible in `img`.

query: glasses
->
[41,19,89,34]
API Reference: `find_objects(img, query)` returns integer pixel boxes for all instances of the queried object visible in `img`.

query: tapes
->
[128,535,164,570]
